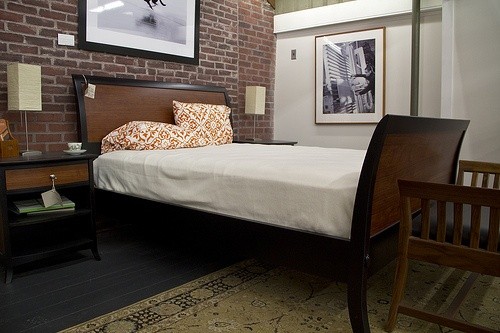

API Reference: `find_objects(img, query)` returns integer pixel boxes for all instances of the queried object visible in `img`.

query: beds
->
[72,74,472,333]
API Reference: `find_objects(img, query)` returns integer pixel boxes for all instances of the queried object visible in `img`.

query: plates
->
[64,149,87,156]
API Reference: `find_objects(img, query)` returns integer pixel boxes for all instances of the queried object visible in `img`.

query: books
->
[9,193,75,216]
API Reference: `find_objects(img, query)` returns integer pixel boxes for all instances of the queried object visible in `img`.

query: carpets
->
[55,258,500,333]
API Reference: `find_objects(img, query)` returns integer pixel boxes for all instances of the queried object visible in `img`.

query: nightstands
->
[0,152,102,285]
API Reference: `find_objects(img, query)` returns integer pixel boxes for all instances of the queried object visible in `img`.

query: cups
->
[68,142,82,151]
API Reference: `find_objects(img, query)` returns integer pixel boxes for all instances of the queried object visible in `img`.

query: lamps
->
[246,86,267,141]
[7,62,42,157]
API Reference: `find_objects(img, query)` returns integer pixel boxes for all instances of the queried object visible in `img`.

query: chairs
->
[385,161,500,333]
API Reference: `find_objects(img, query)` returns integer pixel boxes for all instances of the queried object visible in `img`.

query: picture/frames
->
[315,26,386,125]
[78,0,200,66]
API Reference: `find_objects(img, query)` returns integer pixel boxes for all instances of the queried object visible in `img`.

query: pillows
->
[100,121,207,154]
[173,100,233,146]
